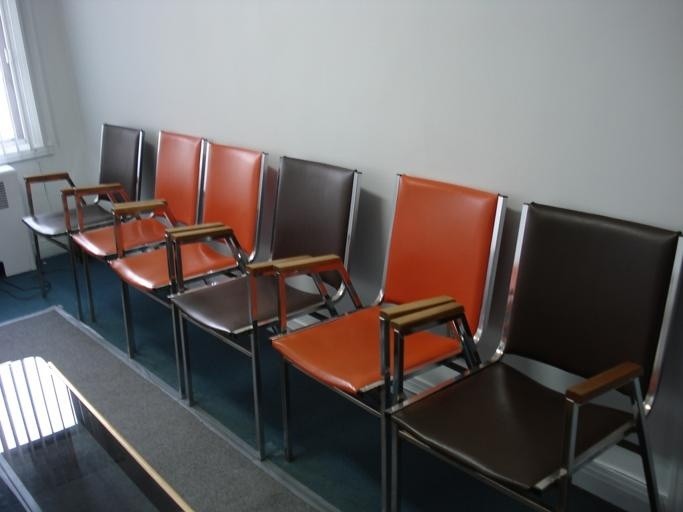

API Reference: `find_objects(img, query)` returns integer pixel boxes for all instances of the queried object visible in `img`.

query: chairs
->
[70,131,205,359]
[270,175,508,512]
[112,140,258,402]
[171,155,361,460]
[21,124,143,320]
[390,202,683,512]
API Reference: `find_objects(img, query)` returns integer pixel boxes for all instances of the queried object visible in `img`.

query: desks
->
[0,356,196,511]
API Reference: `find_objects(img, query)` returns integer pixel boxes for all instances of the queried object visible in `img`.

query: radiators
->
[0,164,38,278]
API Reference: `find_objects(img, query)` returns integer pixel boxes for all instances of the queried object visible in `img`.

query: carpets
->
[0,305,340,511]
